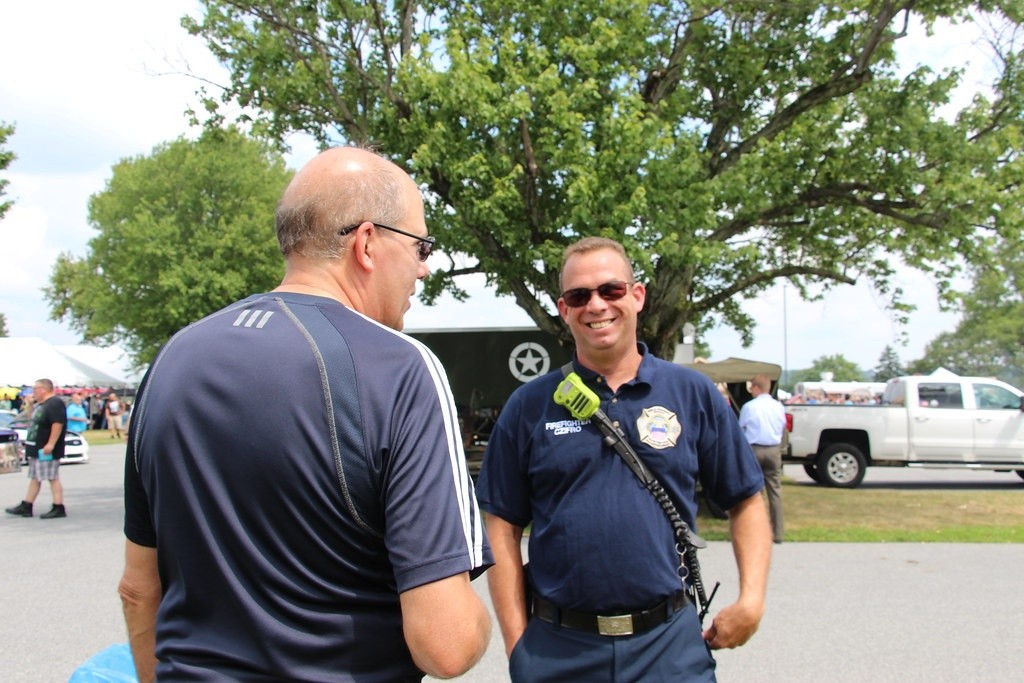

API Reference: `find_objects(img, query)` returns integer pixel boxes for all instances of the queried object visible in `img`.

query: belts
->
[525,590,688,637]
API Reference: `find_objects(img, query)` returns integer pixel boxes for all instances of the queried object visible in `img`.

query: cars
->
[0,409,88,464]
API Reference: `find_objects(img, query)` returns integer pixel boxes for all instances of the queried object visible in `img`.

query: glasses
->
[559,280,634,307]
[338,222,436,262]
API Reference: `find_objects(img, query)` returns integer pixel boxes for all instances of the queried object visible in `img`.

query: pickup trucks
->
[783,375,1024,488]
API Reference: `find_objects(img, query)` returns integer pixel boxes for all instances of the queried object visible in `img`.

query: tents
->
[682,357,781,417]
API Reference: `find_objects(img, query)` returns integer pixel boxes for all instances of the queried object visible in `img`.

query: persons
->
[0,393,131,438]
[795,388,881,406]
[4,379,67,519]
[475,237,771,683]
[65,394,88,432]
[116,147,495,683]
[741,376,785,544]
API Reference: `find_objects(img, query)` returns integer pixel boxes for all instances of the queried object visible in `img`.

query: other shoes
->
[40,504,66,519]
[4,502,33,517]
